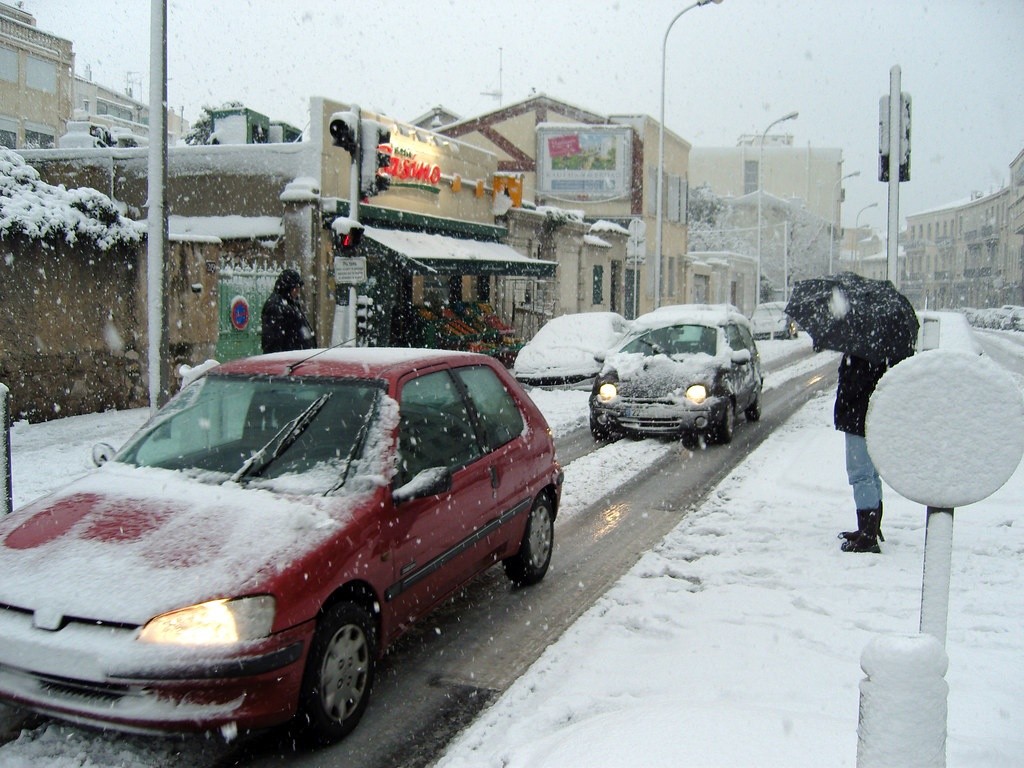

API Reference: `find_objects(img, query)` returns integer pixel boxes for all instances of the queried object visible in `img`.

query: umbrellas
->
[784,272,920,366]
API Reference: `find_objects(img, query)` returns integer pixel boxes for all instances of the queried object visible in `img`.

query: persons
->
[835,353,885,553]
[261,268,317,352]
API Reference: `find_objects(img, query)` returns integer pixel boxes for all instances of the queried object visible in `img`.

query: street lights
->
[853,202,879,272]
[756,109,802,307]
[653,0,724,310]
[829,171,863,276]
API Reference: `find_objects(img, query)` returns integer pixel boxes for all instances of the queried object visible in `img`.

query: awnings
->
[361,227,558,279]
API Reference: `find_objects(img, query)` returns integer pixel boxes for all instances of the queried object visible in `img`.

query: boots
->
[841,508,880,553]
[838,501,885,542]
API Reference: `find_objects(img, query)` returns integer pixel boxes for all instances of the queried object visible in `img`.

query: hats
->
[277,269,304,289]
[428,304,443,318]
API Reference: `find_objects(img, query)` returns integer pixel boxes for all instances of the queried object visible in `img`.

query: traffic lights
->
[336,217,365,259]
[328,118,353,160]
[364,118,393,196]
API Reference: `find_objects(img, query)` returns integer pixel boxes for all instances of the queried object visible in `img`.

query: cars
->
[513,311,628,386]
[0,346,564,753]
[587,311,764,444]
[749,301,800,341]
